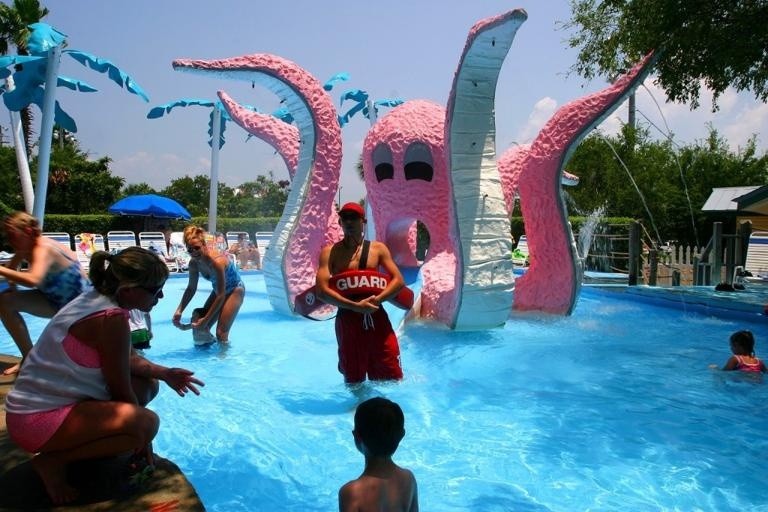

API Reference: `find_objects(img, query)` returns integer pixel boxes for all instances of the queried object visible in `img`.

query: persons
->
[2,246,208,504]
[171,308,218,353]
[229,234,263,271]
[337,399,419,509]
[173,224,247,342]
[0,209,91,376]
[312,203,405,381]
[706,331,767,372]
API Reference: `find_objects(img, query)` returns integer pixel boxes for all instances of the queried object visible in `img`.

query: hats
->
[339,203,365,217]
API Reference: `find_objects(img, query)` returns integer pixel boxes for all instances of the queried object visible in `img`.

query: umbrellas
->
[107,194,193,233]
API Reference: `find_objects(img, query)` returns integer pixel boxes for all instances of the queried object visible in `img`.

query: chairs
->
[511,234,531,269]
[730,230,767,290]
[38,232,274,273]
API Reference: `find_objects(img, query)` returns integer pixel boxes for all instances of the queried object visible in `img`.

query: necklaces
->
[337,234,366,263]
